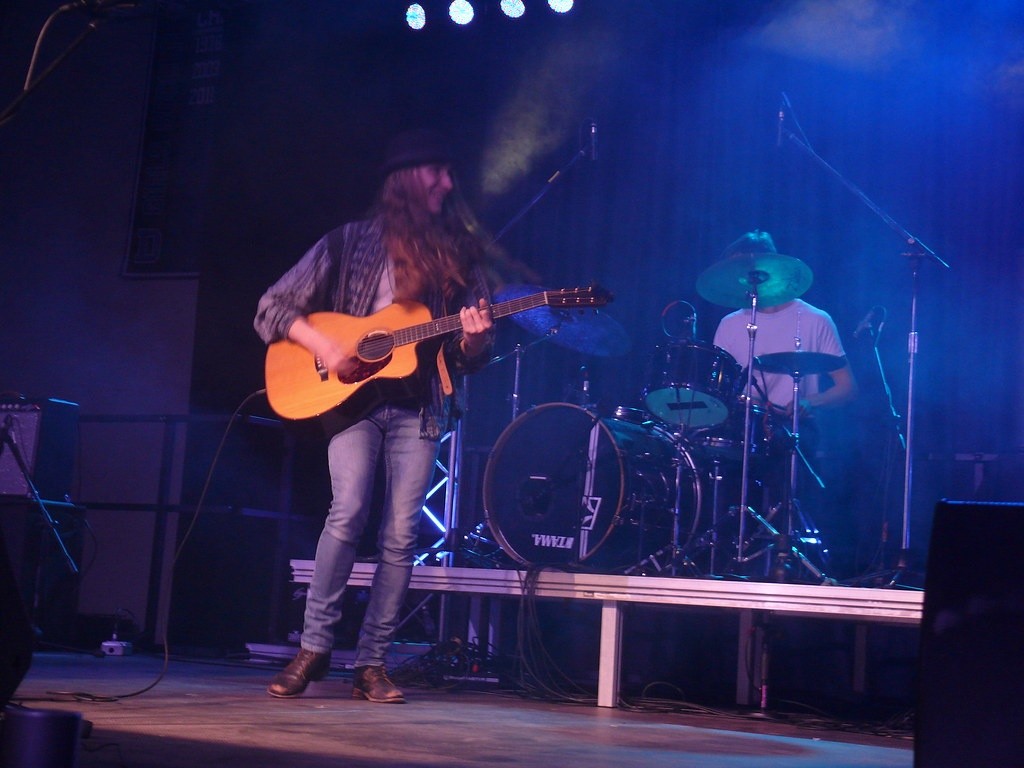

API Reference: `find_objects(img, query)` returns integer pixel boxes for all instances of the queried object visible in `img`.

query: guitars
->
[265,285,614,438]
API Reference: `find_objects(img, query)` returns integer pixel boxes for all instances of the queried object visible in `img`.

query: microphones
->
[692,312,697,339]
[592,123,600,161]
[854,307,877,339]
[776,100,786,147]
[61,0,142,10]
[737,358,758,393]
[0,415,9,454]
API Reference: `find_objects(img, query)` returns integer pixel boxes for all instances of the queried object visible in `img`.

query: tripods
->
[622,126,950,588]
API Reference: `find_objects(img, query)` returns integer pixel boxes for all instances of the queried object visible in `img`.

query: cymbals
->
[694,251,816,307]
[489,285,631,358]
[749,351,849,377]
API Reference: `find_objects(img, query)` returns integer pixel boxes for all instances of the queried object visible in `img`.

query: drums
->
[695,458,778,581]
[641,338,746,427]
[690,404,778,467]
[481,403,703,579]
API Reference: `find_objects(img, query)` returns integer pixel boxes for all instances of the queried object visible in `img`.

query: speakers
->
[0,497,86,652]
[0,397,81,501]
[914,498,1024,768]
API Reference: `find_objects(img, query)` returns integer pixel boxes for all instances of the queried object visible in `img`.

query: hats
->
[376,126,470,186]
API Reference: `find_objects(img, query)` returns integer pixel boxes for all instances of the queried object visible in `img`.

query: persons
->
[711,231,858,422]
[253,131,496,704]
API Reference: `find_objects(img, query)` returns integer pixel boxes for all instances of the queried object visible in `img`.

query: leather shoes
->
[352,665,406,703]
[266,647,331,698]
